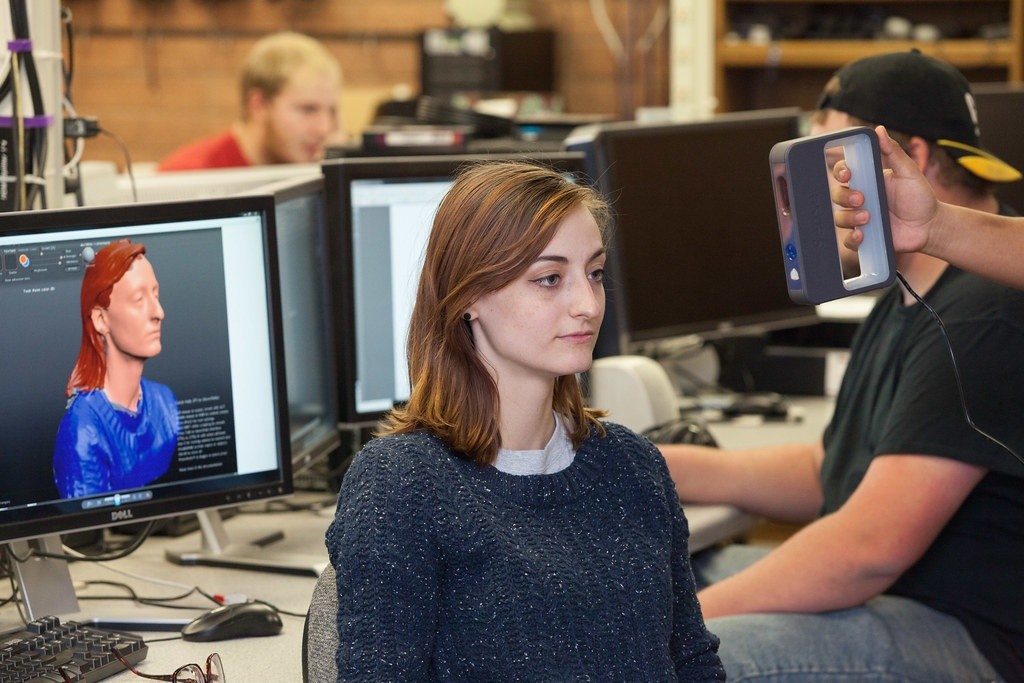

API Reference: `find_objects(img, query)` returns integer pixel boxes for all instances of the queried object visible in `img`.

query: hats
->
[819,47,1021,184]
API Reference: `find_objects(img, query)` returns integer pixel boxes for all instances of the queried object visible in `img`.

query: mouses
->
[180,602,284,643]
[640,417,718,450]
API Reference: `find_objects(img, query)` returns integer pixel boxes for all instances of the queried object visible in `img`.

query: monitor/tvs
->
[0,106,822,627]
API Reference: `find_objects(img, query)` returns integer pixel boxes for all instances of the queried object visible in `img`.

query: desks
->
[0,391,837,683]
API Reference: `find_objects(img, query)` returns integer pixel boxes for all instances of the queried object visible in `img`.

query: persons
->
[49,243,180,500]
[330,164,732,682]
[828,125,1024,290]
[151,31,341,172]
[653,41,1023,682]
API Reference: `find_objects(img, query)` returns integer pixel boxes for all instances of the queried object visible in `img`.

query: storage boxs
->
[418,26,553,90]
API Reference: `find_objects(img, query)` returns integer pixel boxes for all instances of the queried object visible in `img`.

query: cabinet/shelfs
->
[713,0,1023,115]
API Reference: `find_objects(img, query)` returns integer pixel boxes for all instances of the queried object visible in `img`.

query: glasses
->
[55,646,228,683]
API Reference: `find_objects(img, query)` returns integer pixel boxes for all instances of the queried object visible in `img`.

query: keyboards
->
[0,615,149,683]
